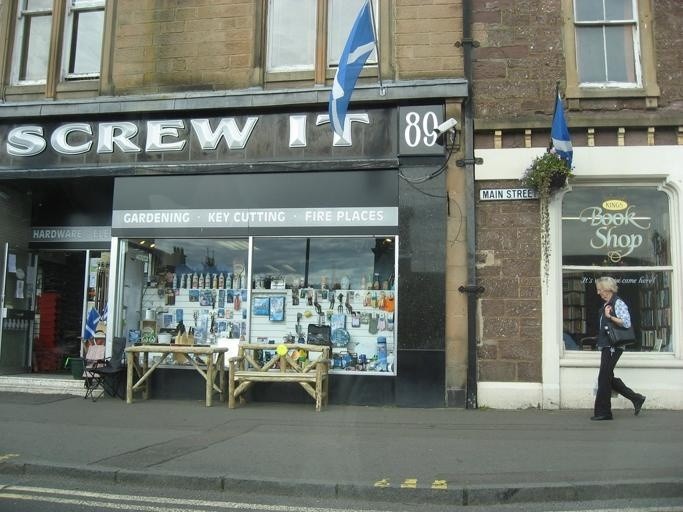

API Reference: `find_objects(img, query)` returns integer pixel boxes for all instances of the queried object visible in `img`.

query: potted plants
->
[521,152,576,287]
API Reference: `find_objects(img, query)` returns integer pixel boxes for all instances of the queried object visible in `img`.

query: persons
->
[79,287,96,335]
[591,276,645,420]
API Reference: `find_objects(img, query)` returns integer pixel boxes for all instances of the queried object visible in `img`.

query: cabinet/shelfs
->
[563,269,588,335]
[637,213,672,349]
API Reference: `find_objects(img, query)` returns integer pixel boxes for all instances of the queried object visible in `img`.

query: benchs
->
[228,343,330,411]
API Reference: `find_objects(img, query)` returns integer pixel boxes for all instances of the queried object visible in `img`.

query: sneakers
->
[634,396,645,415]
[591,414,612,419]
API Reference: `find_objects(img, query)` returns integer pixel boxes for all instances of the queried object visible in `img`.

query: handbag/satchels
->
[605,320,636,346]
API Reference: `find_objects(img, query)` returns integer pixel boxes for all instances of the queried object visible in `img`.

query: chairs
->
[83,337,126,402]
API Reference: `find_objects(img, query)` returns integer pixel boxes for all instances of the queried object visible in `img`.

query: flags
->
[85,309,100,340]
[551,94,573,169]
[329,0,375,137]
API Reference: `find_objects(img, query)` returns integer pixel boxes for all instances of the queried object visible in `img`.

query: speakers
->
[307,323,331,359]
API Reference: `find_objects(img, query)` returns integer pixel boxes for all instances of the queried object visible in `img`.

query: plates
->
[330,326,350,348]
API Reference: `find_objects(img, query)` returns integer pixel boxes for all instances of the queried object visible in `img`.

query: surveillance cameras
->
[433,118,457,136]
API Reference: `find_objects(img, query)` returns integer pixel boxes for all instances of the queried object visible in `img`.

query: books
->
[564,270,673,351]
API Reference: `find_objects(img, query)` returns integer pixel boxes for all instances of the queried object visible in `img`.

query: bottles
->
[172,271,241,289]
[319,312,325,326]
[298,348,305,369]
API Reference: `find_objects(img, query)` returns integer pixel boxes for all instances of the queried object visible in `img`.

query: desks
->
[125,346,228,406]
